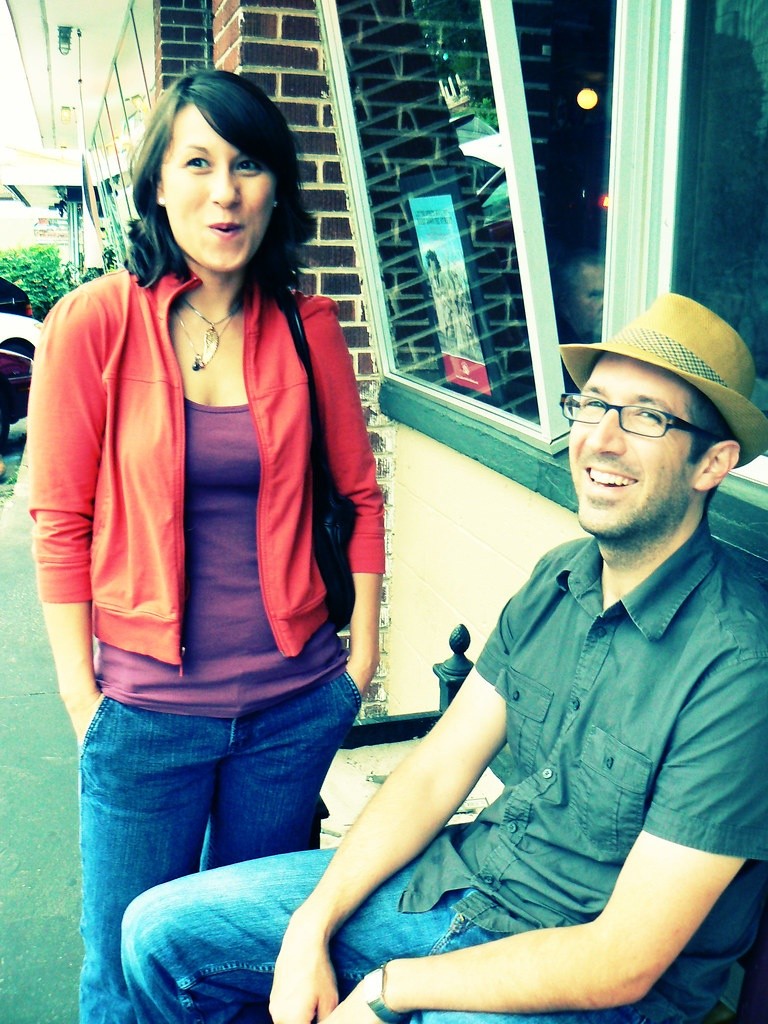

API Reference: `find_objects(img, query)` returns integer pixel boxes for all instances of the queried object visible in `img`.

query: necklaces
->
[176,296,242,371]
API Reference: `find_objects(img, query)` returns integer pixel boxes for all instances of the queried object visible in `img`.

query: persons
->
[25,70,383,1024]
[122,293,768,1024]
[553,250,604,343]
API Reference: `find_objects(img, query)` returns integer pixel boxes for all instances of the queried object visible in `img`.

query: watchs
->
[364,965,412,1024]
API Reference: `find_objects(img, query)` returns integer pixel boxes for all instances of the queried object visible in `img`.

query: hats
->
[558,293,768,468]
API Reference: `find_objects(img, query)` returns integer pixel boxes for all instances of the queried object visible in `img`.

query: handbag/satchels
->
[275,282,358,633]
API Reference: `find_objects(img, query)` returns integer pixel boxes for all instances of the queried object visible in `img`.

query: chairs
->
[309,623,768,1024]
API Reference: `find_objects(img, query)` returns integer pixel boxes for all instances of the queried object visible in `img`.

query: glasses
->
[561,392,720,442]
